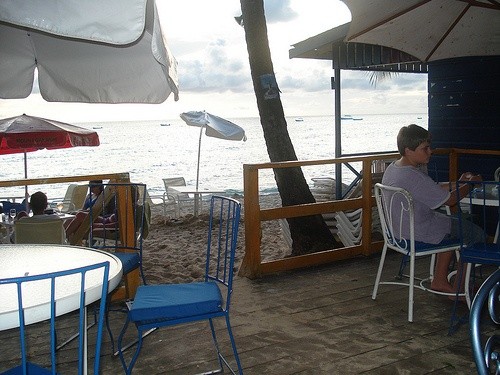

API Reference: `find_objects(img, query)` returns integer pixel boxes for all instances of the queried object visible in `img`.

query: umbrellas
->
[342,0,500,63]
[1,0,181,103]
[178,109,248,191]
[0,114,99,213]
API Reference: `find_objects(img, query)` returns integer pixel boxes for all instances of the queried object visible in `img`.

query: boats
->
[296,117,303,121]
[341,113,363,120]
[417,117,422,119]
[160,123,170,126]
[93,127,102,129]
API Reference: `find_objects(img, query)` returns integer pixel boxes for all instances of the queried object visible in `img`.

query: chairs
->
[0,158,500,375]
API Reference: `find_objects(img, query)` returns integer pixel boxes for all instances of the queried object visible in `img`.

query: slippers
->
[432,292,475,304]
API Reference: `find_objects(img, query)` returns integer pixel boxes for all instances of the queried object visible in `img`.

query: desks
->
[0,243,122,331]
[447,197,500,282]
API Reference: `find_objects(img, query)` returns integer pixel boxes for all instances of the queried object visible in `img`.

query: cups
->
[373,159,386,173]
[0,213,5,222]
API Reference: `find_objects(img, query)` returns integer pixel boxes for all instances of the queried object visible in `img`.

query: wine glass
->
[57,202,64,213]
[10,209,16,221]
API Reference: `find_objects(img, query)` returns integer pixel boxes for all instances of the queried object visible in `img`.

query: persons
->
[381,125,483,303]
[9,191,66,246]
[63,180,139,239]
